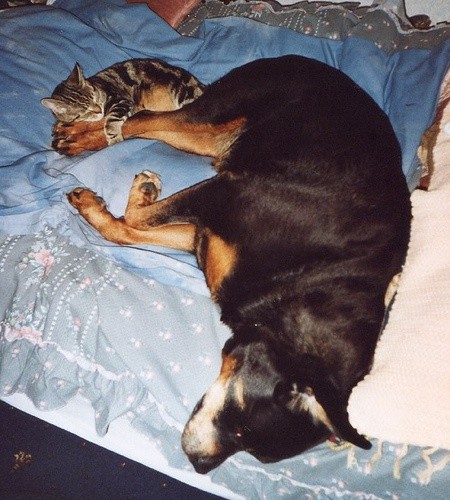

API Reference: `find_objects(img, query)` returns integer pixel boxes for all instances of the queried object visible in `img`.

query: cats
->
[41,56,207,146]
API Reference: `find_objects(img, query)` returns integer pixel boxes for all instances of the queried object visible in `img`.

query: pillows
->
[148,1,202,28]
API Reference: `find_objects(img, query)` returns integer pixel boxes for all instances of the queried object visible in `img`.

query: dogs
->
[50,54,413,475]
